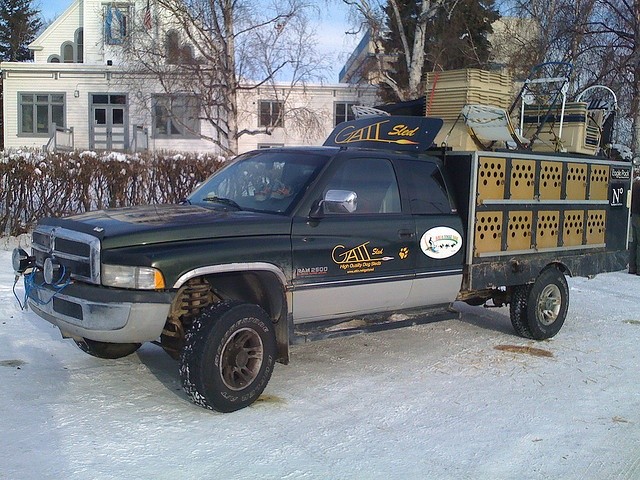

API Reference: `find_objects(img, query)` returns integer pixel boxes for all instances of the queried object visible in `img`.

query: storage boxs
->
[422,70,515,151]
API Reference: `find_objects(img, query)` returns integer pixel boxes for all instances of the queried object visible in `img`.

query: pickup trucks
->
[14,117,639,413]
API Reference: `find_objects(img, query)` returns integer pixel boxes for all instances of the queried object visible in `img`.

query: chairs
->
[442,63,574,153]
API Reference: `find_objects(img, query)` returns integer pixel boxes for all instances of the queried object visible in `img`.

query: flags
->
[143,0,153,29]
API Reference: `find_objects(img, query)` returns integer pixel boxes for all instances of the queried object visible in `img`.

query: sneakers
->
[271,181,294,200]
[254,182,271,202]
